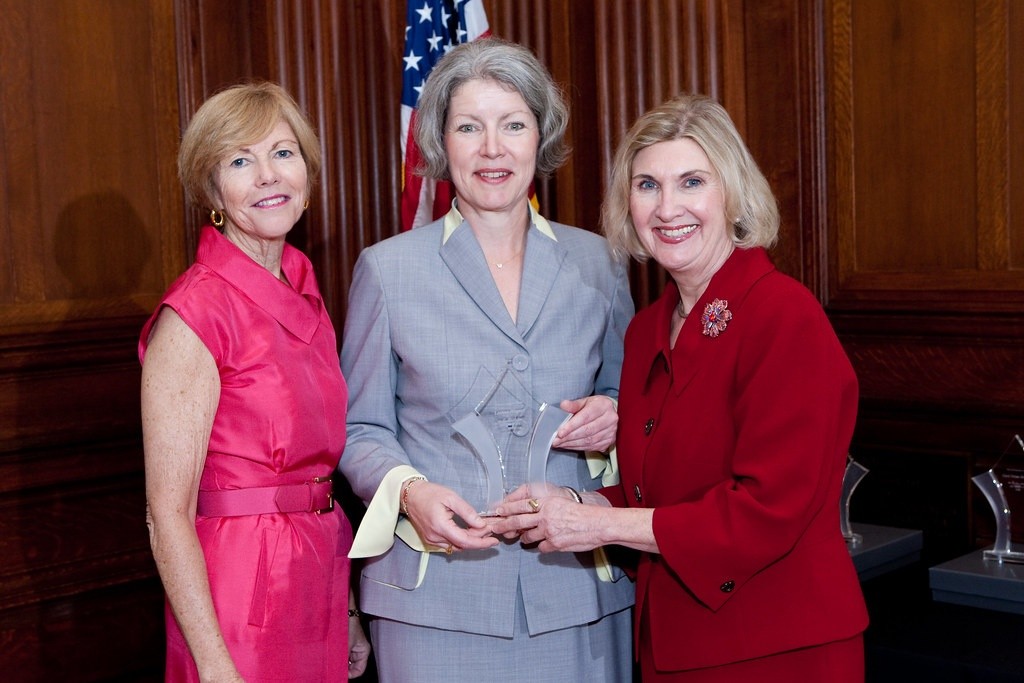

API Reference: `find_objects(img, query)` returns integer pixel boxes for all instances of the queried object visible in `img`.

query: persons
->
[337,36,636,683]
[493,91,869,683]
[137,84,371,683]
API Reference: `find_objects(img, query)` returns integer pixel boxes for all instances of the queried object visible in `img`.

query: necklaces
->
[496,253,521,268]
[677,299,690,318]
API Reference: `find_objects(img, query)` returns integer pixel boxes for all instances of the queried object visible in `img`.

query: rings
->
[348,660,351,664]
[530,498,538,512]
[447,544,453,554]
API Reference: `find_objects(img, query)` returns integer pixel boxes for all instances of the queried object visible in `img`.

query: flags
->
[401,0,539,232]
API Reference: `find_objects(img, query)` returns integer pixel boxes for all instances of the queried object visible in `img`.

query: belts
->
[196,476,334,517]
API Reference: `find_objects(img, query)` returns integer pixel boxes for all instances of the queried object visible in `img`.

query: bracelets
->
[562,485,583,503]
[402,477,423,517]
[348,609,359,617]
